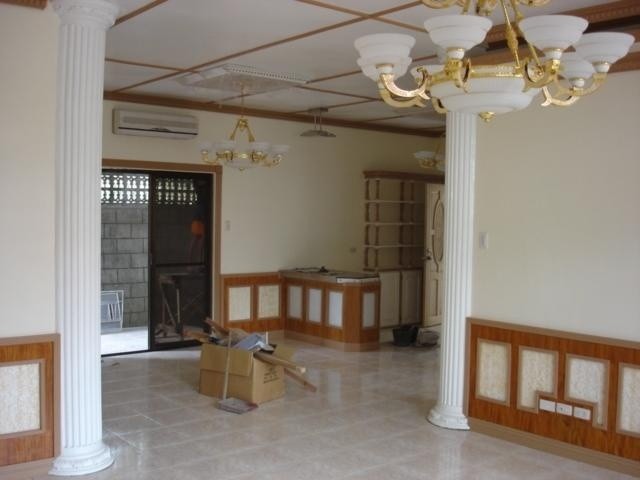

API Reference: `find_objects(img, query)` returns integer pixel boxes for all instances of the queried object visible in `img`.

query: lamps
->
[300,108,337,139]
[199,97,291,177]
[353,0,636,123]
[413,131,446,172]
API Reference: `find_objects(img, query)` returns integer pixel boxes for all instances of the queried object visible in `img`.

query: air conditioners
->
[112,106,200,140]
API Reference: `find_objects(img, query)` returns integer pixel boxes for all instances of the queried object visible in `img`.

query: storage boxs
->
[199,326,297,405]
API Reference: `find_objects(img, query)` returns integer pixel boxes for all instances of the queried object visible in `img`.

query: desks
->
[156,273,211,337]
